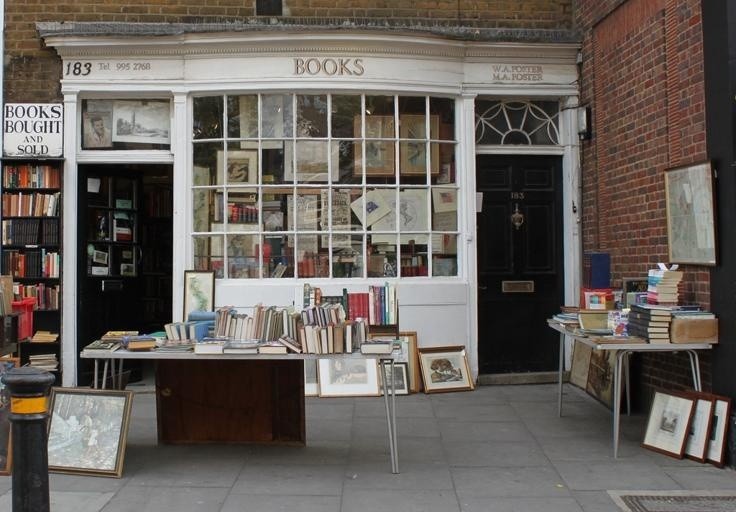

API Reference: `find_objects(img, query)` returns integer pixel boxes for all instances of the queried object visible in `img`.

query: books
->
[210,206,426,279]
[550,263,718,345]
[301,283,398,354]
[85,302,302,354]
[1,159,59,374]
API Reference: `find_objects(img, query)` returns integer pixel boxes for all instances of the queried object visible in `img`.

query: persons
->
[84,115,113,147]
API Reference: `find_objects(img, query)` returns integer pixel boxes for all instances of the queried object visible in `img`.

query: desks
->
[81,337,399,474]
[549,319,716,460]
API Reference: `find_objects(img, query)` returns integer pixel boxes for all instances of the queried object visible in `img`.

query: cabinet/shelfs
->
[79,164,172,349]
[2,158,65,477]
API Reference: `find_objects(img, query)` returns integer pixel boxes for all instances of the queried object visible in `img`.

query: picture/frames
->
[0,358,20,475]
[183,270,215,322]
[662,158,720,269]
[304,331,476,398]
[640,389,731,469]
[46,387,132,480]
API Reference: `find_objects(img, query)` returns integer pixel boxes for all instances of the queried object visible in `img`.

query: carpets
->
[606,489,734,512]
